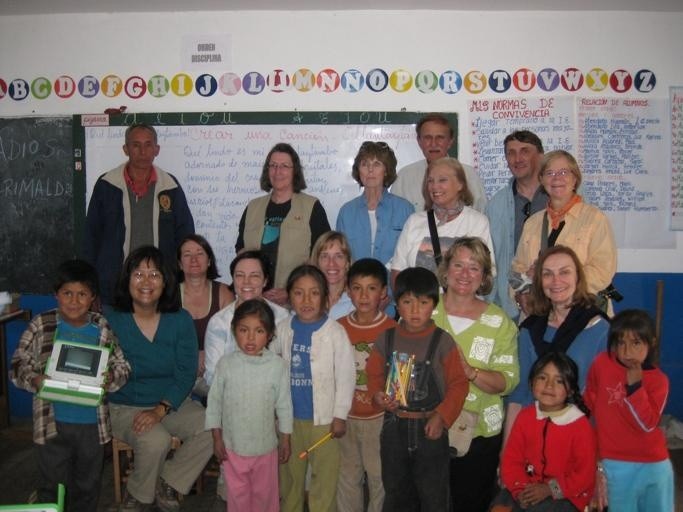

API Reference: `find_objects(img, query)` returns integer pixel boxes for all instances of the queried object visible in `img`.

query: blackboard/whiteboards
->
[0,112,458,295]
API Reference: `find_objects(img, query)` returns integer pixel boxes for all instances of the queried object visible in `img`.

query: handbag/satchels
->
[447,409,479,458]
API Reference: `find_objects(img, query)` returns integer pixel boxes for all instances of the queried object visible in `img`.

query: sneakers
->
[155,476,181,512]
[117,486,145,512]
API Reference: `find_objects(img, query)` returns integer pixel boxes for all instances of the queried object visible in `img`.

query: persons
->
[103,231,673,512]
[487,131,550,317]
[388,115,488,214]
[507,149,616,325]
[391,157,497,295]
[336,142,415,264]
[83,124,194,307]
[235,143,331,306]
[6,261,129,512]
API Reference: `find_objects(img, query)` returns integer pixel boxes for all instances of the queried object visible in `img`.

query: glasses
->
[134,272,161,281]
[543,169,572,176]
[266,163,293,171]
[361,141,389,150]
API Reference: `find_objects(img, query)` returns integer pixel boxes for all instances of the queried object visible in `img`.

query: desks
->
[0,309,31,429]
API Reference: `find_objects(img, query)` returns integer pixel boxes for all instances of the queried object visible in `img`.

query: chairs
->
[111,437,181,502]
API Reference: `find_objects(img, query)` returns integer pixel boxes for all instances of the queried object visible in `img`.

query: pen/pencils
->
[299,432,332,460]
[384,351,416,408]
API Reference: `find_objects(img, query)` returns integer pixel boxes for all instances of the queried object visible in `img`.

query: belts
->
[391,408,433,420]
